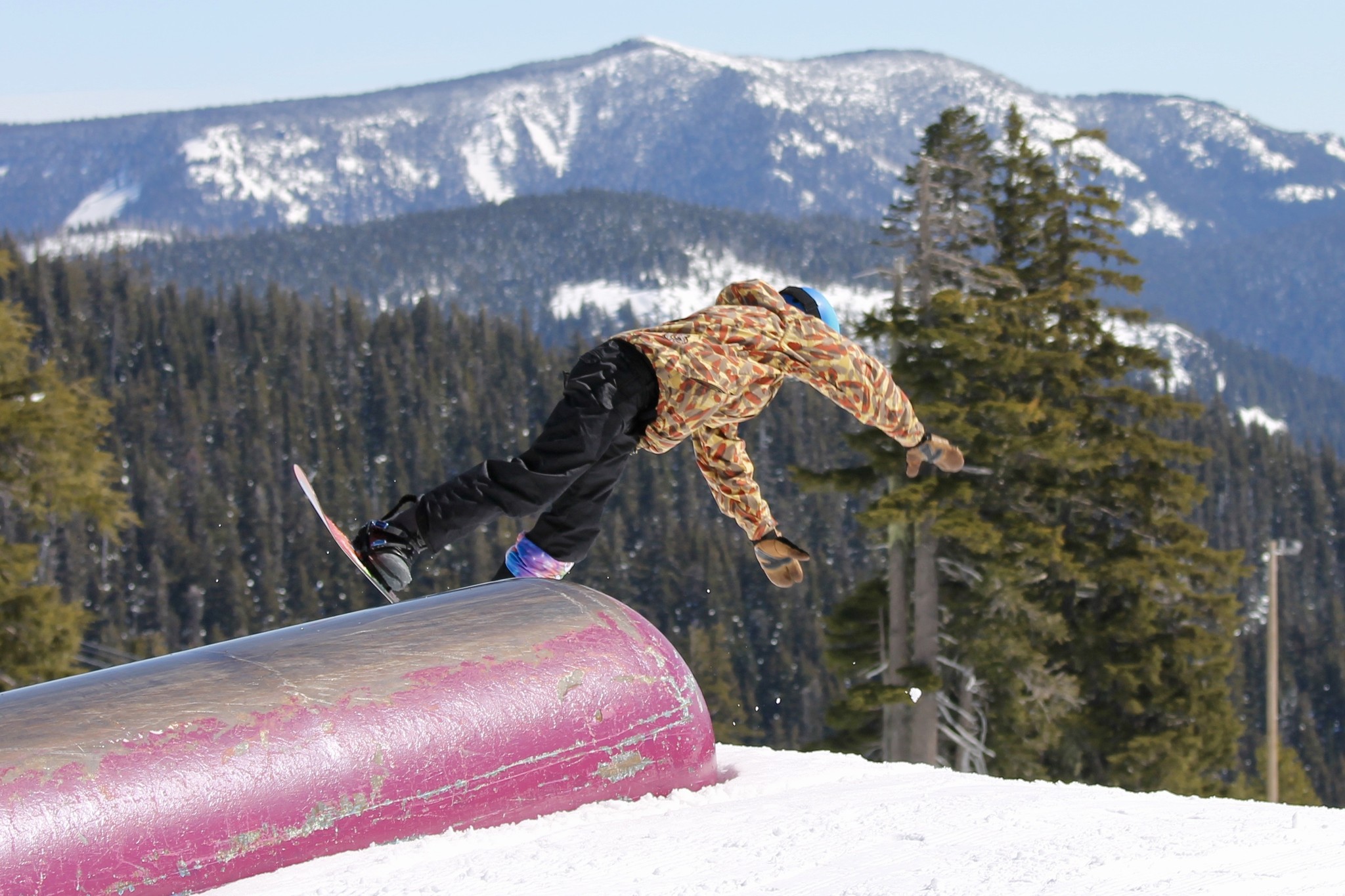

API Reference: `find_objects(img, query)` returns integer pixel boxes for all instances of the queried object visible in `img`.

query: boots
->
[370,503,427,592]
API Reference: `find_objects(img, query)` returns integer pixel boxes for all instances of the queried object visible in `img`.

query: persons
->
[350,279,966,596]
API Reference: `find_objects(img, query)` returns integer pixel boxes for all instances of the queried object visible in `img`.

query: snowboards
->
[292,460,400,607]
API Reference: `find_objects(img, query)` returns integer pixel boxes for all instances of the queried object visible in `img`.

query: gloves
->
[752,528,810,587]
[906,433,964,478]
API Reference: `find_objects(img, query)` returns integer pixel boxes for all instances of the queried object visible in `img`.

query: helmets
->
[781,287,839,333]
